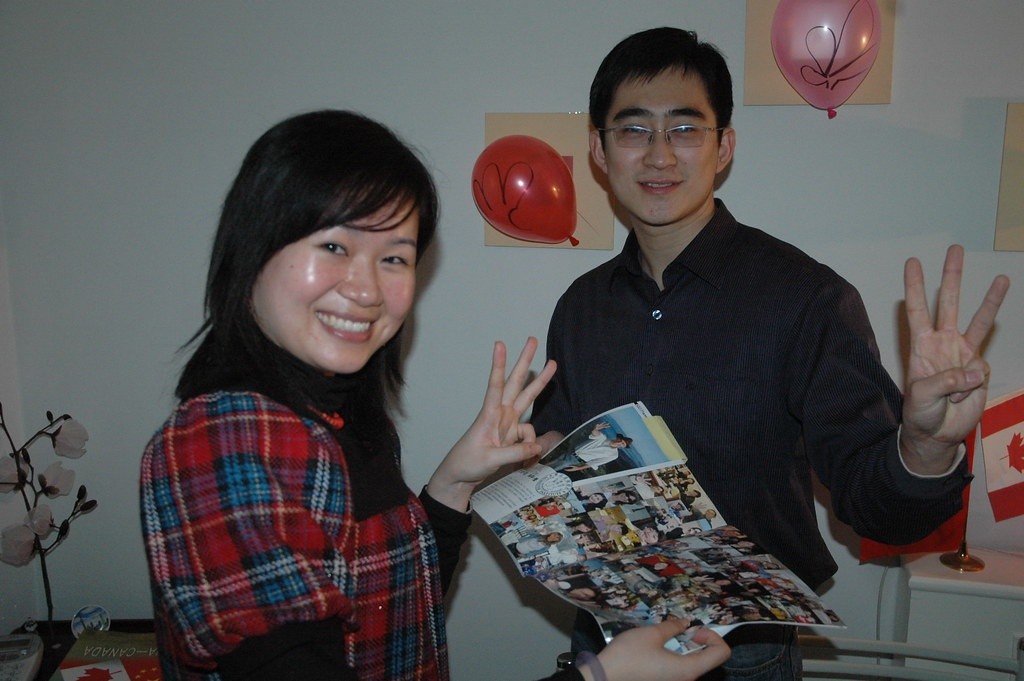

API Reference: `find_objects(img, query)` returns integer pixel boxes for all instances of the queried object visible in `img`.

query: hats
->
[616,433,633,448]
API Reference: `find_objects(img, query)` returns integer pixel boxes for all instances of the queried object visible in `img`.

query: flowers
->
[0,402,98,628]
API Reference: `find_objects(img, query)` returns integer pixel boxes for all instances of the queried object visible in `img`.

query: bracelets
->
[576,650,607,681]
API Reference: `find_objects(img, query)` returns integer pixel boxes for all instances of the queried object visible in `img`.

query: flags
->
[981,389,1024,522]
[859,425,977,558]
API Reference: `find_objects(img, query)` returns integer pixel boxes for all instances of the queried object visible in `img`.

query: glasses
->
[597,126,724,147]
[626,492,632,502]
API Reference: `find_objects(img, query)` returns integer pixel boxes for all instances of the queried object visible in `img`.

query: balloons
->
[770,0,882,118]
[471,135,580,247]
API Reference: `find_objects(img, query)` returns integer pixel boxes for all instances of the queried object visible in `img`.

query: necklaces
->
[306,406,346,427]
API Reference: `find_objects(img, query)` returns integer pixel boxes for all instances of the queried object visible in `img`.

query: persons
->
[529,28,1010,681]
[491,421,841,653]
[140,110,733,681]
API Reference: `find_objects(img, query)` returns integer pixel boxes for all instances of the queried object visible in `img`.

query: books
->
[50,628,164,681]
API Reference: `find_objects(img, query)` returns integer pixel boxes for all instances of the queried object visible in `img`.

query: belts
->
[571,448,588,464]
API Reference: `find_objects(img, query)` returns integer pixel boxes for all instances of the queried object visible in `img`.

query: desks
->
[904,546,1024,680]
[9,618,156,681]
